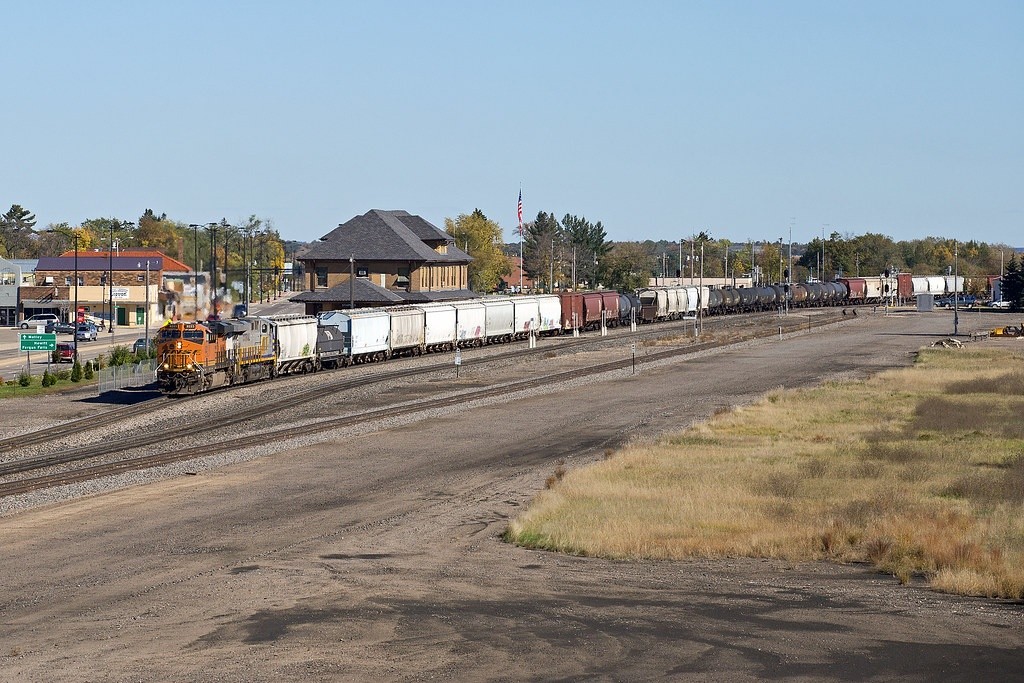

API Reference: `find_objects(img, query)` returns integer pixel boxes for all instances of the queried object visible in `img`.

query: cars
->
[48,322,77,335]
[77,324,97,341]
[934,291,977,307]
[988,298,1012,307]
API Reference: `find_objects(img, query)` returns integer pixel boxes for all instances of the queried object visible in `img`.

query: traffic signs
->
[19,331,58,353]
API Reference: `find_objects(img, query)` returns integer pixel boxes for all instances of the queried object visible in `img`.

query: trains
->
[148,275,971,397]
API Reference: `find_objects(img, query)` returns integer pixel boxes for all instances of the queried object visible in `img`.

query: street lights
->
[188,223,268,318]
[107,220,132,333]
[44,228,81,361]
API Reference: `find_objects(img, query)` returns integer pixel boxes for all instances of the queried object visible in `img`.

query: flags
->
[518,189,523,236]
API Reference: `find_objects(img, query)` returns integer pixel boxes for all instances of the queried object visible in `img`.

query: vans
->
[18,311,58,329]
